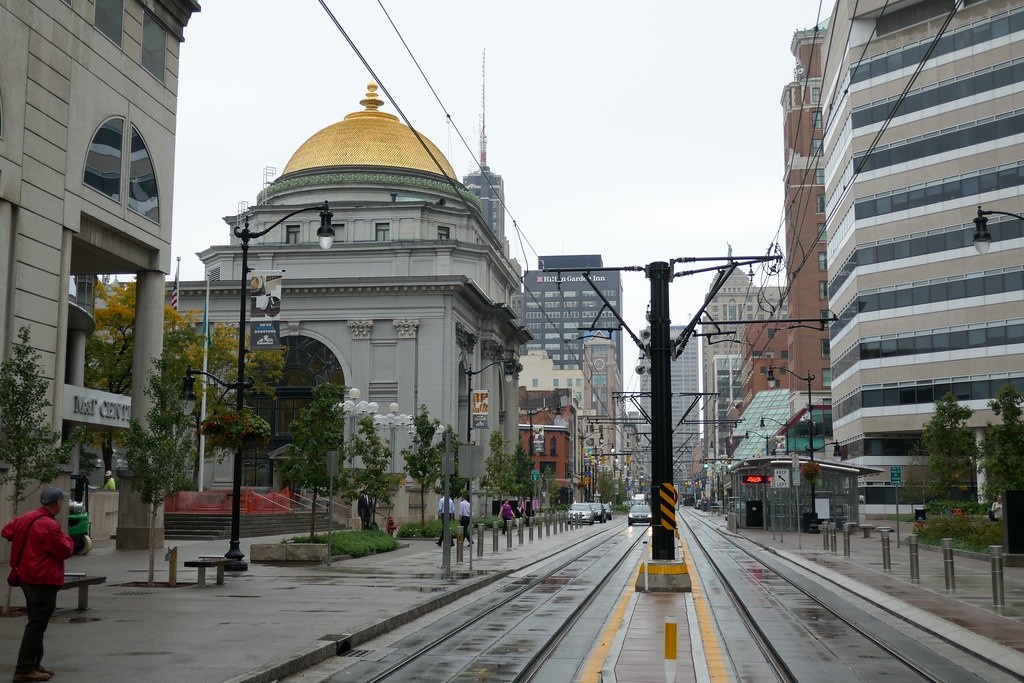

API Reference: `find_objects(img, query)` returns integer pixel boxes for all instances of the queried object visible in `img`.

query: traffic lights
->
[695,479,699,486]
[684,481,688,487]
[727,457,733,469]
[704,457,709,470]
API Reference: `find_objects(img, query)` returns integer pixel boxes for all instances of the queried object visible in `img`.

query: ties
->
[364,495,367,504]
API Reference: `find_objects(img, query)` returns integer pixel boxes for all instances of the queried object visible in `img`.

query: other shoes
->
[451,544,455,546]
[38,666,55,675]
[13,670,50,681]
[436,541,441,546]
[468,542,474,547]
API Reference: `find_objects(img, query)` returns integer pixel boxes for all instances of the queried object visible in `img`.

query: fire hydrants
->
[384,515,397,536]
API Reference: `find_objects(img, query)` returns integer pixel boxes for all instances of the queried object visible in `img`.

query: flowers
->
[801,460,820,476]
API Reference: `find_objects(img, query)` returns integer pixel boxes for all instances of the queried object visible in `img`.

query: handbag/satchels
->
[7,569,21,587]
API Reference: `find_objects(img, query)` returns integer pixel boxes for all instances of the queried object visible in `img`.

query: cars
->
[694,500,701,510]
[566,502,596,525]
[627,505,652,527]
[602,503,614,520]
[587,502,607,524]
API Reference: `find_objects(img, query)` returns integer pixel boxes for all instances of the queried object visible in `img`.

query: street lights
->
[759,416,792,454]
[745,430,771,456]
[465,358,516,503]
[524,403,563,528]
[765,361,842,533]
[580,431,606,504]
[331,387,380,486]
[372,401,419,476]
[591,441,615,503]
[176,198,340,572]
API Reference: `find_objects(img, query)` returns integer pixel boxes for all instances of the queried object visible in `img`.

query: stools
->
[845,522,858,534]
[861,525,873,538]
[878,527,891,542]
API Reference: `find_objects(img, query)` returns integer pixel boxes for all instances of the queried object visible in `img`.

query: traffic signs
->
[774,469,790,488]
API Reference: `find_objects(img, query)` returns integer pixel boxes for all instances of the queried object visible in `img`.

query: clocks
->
[594,359,605,370]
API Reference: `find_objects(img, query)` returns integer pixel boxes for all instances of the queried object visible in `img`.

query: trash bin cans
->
[915,509,926,522]
[804,512,818,533]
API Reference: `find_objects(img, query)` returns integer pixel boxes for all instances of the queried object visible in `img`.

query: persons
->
[0,486,76,683]
[497,499,515,535]
[459,494,474,546]
[513,502,528,535]
[435,491,456,547]
[991,495,1003,521]
[357,493,373,530]
[103,471,115,490]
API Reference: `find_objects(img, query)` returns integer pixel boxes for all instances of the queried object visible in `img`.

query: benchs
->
[57,572,107,610]
[185,555,239,587]
